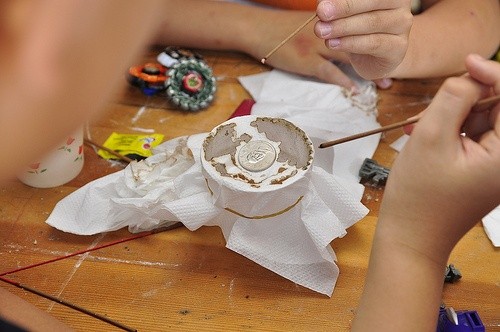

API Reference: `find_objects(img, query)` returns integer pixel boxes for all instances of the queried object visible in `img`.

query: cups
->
[16,124,84,188]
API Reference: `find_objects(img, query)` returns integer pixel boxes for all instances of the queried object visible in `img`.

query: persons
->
[0,0,500,332]
[164,0,500,95]
